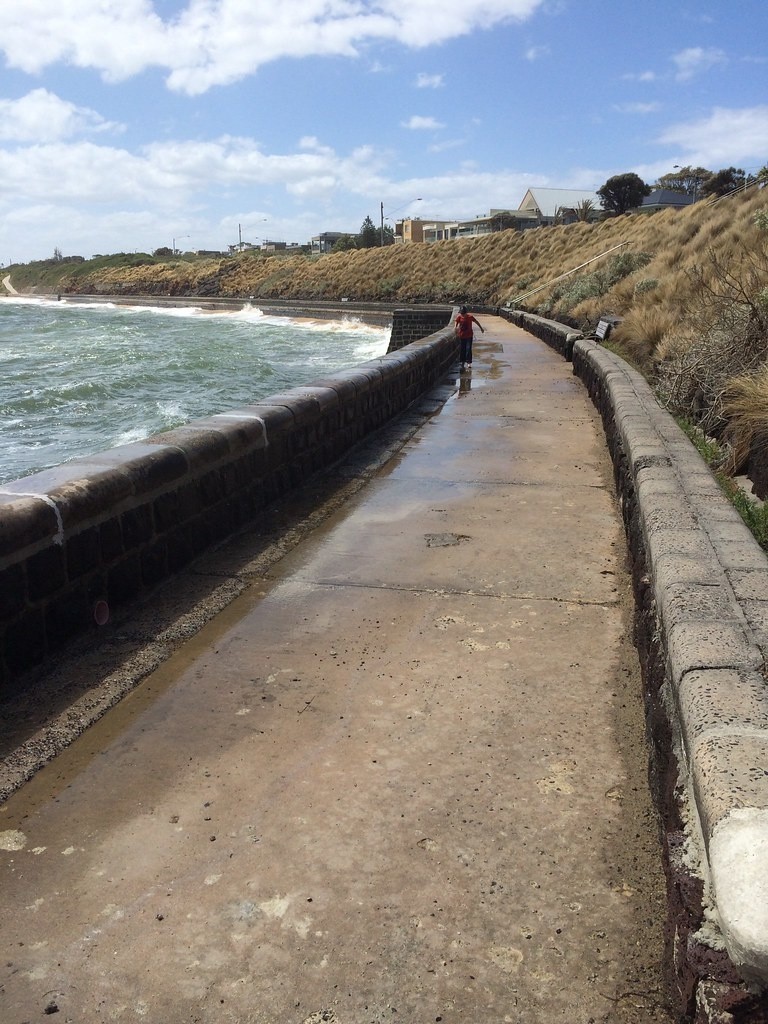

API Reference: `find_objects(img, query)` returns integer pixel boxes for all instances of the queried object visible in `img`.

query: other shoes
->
[468,364,472,367]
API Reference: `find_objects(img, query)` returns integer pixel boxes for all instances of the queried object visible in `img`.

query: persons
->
[453,306,484,368]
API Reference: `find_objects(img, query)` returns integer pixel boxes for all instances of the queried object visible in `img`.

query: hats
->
[459,306,467,313]
